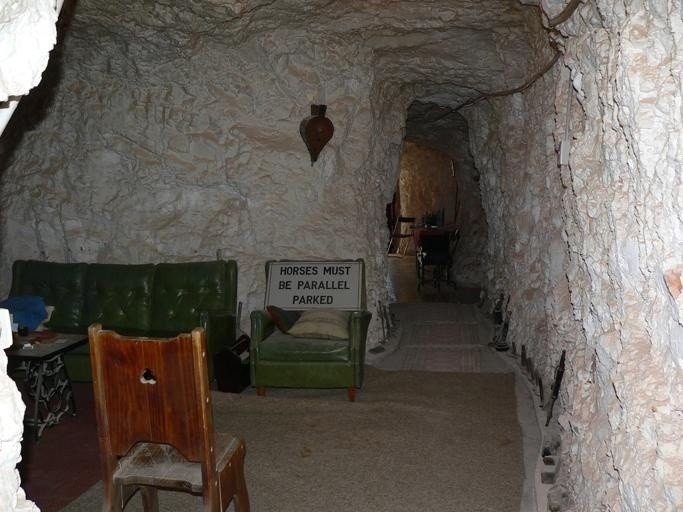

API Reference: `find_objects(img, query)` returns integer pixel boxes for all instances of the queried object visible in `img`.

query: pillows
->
[288,307,351,341]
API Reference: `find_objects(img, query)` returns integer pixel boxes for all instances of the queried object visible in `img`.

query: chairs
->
[85,323,252,512]
[248,259,376,401]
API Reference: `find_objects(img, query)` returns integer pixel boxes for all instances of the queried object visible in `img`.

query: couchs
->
[7,259,236,387]
